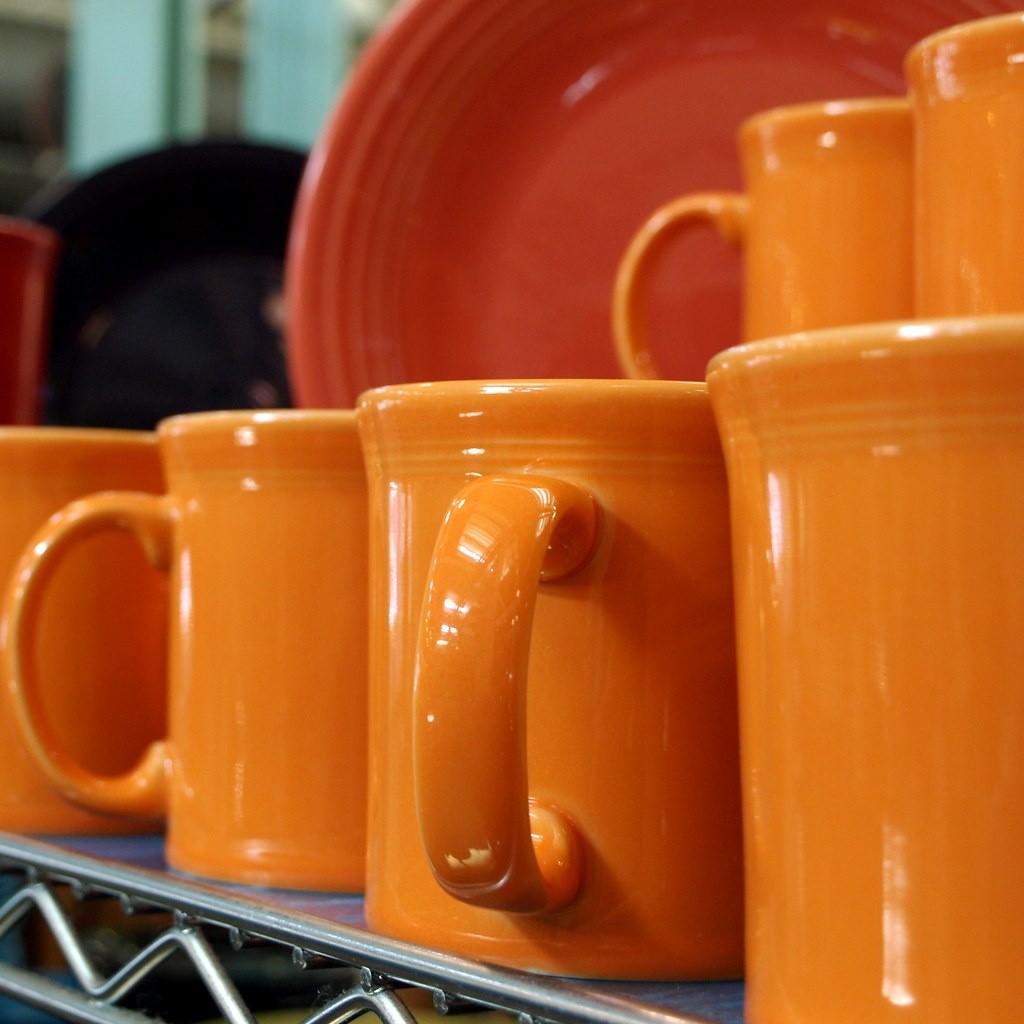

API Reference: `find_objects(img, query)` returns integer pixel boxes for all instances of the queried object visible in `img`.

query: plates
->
[20,140,323,433]
[283,0,1024,425]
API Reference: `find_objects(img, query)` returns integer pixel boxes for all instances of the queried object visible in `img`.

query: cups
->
[606,100,925,394]
[2,422,169,836]
[0,408,365,893]
[704,310,1021,1024]
[354,378,745,985]
[900,11,1020,338]
